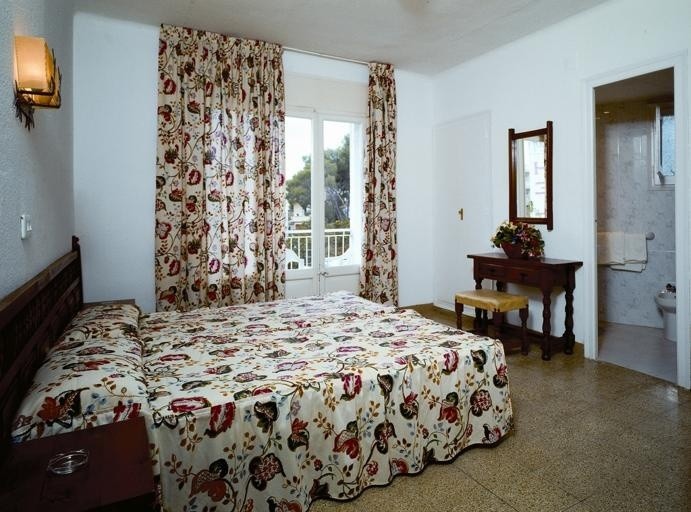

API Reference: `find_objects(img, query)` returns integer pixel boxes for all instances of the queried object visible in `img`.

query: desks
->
[467,250,584,361]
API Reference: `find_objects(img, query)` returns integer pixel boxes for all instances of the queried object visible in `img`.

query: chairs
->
[282,248,304,270]
[324,248,350,266]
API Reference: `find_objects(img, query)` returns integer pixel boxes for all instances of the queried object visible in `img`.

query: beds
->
[1,244,515,510]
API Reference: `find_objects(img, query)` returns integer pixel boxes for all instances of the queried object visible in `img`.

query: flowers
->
[491,219,546,261]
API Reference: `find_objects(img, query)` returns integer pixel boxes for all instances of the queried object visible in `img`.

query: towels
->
[598,231,649,273]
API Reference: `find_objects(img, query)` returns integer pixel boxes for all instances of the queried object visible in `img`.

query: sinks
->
[655,282,677,313]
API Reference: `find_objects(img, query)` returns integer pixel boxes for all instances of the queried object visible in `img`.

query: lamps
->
[11,33,65,132]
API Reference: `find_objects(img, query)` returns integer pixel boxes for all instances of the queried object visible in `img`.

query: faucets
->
[665,284,676,293]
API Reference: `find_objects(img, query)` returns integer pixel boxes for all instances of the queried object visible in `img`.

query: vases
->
[500,242,527,262]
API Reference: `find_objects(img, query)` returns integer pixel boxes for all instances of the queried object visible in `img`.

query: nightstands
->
[0,415,165,511]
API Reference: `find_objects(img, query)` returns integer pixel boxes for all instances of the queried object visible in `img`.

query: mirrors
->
[508,120,555,232]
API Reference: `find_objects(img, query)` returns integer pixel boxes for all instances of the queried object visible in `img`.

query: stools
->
[453,287,530,358]
